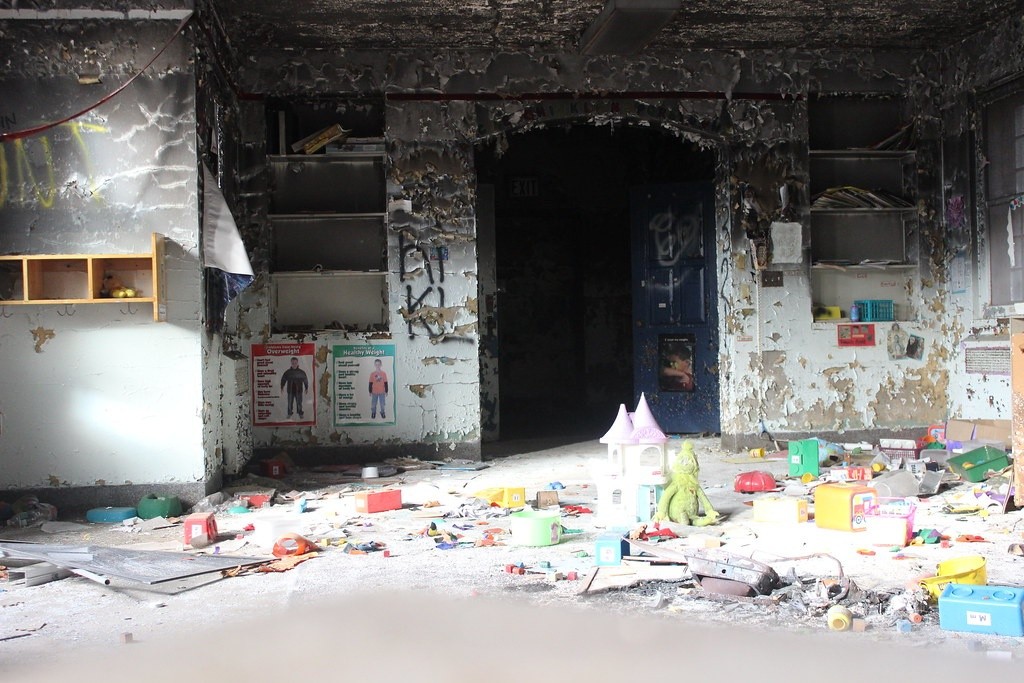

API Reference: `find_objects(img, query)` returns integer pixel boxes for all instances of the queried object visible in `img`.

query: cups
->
[751,448,764,458]
[874,471,919,497]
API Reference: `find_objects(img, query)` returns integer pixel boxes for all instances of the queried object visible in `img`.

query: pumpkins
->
[103,274,136,298]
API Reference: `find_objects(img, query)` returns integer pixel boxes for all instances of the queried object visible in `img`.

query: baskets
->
[854,300,893,321]
[879,441,932,460]
[865,497,917,547]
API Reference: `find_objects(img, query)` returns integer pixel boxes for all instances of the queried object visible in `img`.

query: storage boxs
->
[537,491,559,510]
[814,418,1012,546]
[594,531,630,566]
[688,535,720,549]
[938,584,1024,636]
[752,498,807,524]
[854,300,895,322]
[354,488,402,513]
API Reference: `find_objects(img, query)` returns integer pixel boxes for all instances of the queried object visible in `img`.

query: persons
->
[280,357,309,419]
[368,359,389,420]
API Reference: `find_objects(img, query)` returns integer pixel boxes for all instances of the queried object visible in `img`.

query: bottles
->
[7,508,49,528]
[850,305,859,322]
[827,605,852,631]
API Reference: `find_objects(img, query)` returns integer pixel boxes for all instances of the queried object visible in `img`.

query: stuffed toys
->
[654,439,719,526]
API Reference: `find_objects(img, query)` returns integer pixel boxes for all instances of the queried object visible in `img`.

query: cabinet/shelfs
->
[266,150,391,341]
[808,148,919,268]
[0,232,167,323]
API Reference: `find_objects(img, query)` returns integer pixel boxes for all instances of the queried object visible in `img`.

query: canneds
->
[850,305,859,322]
